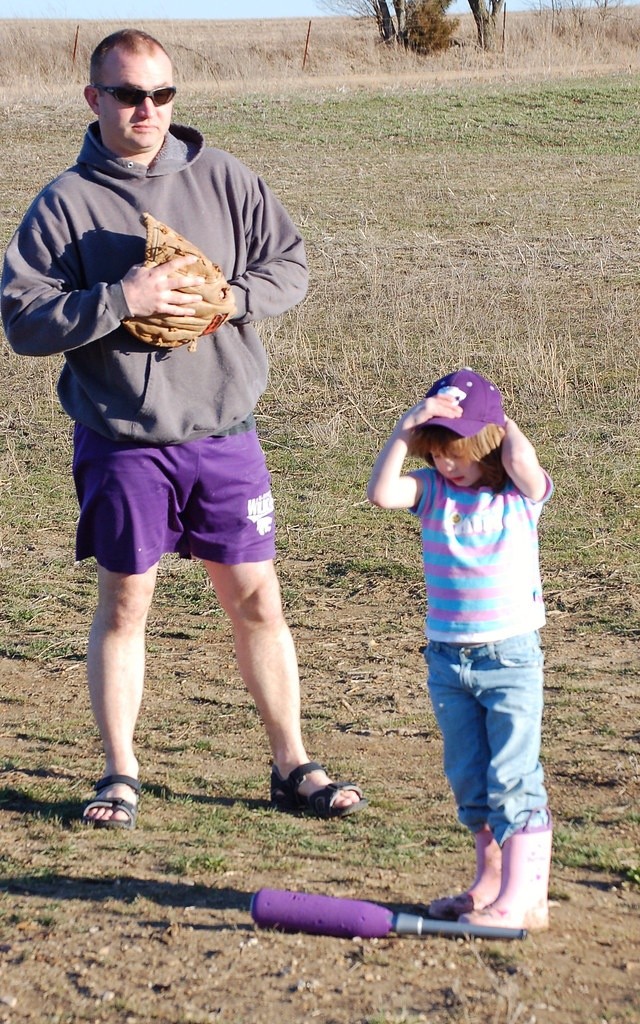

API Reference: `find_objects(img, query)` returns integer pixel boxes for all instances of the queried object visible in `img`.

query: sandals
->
[271,763,367,816]
[83,775,142,829]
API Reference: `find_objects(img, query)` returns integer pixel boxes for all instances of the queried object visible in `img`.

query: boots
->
[428,823,502,920]
[459,805,549,930]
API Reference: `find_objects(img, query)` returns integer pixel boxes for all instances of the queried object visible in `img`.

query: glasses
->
[90,82,176,107]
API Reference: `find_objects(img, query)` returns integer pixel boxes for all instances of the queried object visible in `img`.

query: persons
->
[1,28,367,829]
[371,369,557,935]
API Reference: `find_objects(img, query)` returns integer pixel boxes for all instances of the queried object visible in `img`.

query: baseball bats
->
[250,884,529,943]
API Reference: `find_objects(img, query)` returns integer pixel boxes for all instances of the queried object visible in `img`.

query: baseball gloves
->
[119,209,237,355]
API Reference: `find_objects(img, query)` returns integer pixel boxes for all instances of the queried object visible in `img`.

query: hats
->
[415,366,505,437]
